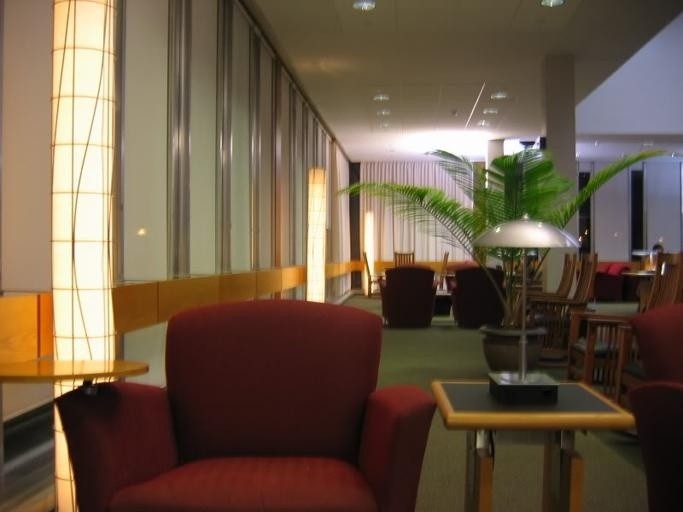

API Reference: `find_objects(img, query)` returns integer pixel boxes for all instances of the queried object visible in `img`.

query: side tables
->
[0,356,149,387]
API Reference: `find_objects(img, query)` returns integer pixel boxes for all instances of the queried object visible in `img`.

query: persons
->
[648,245,664,267]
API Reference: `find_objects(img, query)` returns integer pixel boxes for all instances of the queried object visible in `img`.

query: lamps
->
[473,214,581,403]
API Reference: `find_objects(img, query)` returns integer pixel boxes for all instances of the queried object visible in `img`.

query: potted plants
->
[329,142,681,373]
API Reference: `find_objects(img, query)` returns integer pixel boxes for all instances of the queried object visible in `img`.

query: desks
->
[430,378,638,508]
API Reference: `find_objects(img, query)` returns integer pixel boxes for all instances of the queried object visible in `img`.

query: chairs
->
[624,305,681,510]
[363,251,680,331]
[56,299,435,511]
[569,252,681,405]
